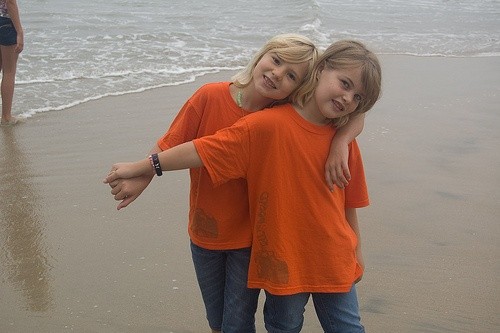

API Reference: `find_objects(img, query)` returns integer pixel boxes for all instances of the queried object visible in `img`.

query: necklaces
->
[236,87,245,109]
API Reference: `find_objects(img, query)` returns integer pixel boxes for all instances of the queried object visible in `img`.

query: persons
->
[101,39,381,333]
[0,0,25,127]
[108,33,364,333]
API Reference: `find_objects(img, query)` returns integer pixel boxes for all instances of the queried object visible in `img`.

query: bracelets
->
[149,154,163,177]
